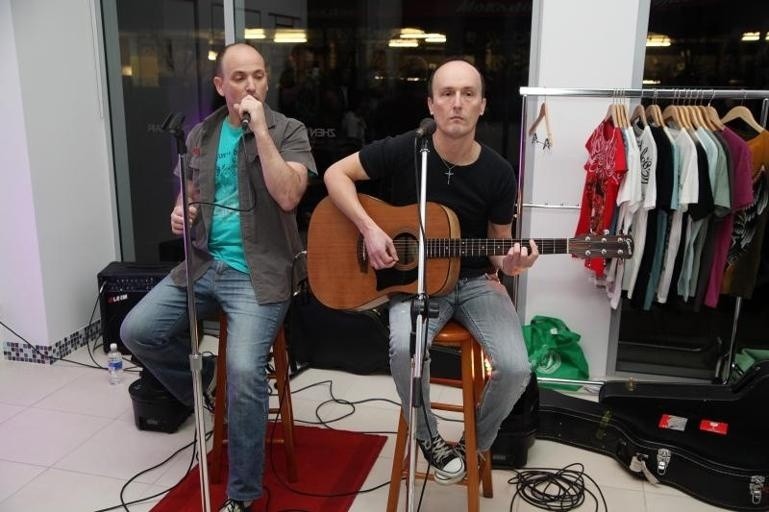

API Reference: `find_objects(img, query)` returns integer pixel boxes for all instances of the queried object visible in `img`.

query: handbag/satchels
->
[518,314,591,392]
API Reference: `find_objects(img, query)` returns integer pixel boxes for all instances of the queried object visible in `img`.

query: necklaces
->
[434,136,472,184]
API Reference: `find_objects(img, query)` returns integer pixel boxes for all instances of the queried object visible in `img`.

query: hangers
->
[603,88,764,134]
[527,86,554,152]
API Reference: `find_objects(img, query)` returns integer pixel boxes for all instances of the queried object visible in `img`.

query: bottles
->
[107,343,123,385]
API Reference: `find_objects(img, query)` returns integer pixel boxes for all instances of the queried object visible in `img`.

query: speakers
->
[97,261,204,356]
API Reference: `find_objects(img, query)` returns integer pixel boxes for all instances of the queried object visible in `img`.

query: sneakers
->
[204,355,228,426]
[416,431,469,486]
[217,498,256,511]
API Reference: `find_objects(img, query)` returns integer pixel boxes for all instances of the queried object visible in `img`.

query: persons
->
[323,53,541,485]
[118,39,323,512]
[278,44,525,173]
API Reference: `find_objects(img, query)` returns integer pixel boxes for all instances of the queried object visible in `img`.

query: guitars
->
[306,192,635,312]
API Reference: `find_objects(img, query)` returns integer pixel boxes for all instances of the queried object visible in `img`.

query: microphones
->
[239,111,251,128]
[415,118,437,139]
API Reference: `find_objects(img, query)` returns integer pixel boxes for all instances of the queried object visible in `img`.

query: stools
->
[210,307,299,483]
[387,320,494,512]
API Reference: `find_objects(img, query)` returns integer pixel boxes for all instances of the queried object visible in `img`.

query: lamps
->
[742,31,768,43]
[645,34,674,49]
[246,27,447,48]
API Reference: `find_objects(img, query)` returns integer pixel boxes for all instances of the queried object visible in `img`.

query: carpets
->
[148,420,388,512]
